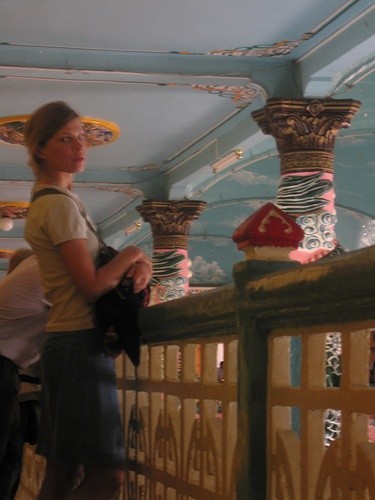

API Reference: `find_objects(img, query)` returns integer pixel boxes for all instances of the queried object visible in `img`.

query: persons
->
[24,101,152,500]
[0,248,46,500]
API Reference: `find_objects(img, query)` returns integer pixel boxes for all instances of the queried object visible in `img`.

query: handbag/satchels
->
[93,246,150,368]
